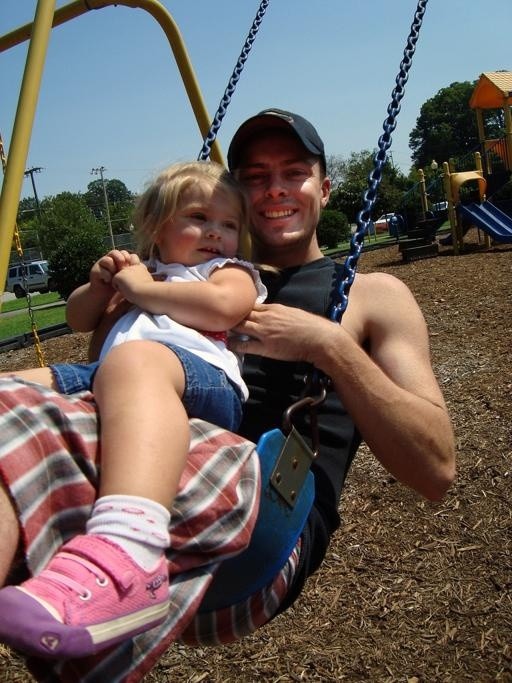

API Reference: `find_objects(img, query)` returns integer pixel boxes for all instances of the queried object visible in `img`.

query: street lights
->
[430,159,441,210]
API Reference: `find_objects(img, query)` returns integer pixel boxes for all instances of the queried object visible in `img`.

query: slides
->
[461,197,512,241]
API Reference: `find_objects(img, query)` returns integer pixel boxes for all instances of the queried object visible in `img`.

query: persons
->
[1,159,269,659]
[0,107,456,682]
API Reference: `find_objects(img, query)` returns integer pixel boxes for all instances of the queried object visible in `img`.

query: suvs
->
[6,258,58,298]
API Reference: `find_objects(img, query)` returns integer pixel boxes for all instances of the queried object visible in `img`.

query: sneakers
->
[1,531,172,657]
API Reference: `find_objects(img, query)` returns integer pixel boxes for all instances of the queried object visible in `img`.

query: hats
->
[227,107,324,171]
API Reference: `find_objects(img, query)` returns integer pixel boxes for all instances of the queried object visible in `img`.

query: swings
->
[195,2,426,609]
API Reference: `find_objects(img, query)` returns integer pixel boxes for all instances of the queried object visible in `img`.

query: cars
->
[375,212,396,233]
[433,200,450,211]
